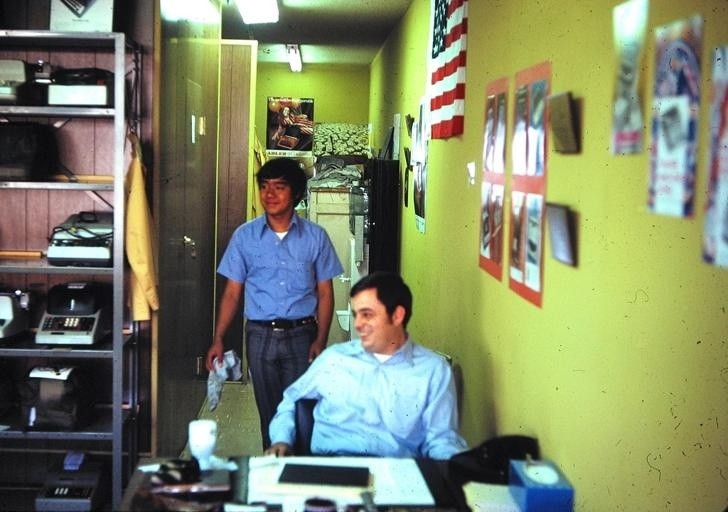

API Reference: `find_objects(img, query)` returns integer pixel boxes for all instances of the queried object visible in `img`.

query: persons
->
[205,158,345,455]
[265,271,471,461]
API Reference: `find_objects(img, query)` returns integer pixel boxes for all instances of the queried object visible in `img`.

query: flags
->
[430,0,467,138]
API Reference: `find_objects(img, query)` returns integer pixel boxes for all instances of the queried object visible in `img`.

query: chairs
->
[292,395,319,452]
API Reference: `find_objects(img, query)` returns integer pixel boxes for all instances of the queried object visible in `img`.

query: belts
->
[243,315,317,333]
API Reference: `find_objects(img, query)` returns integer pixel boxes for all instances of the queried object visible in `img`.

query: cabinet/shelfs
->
[0,27,135,512]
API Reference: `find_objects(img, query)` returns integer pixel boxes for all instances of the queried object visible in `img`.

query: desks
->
[120,453,576,510]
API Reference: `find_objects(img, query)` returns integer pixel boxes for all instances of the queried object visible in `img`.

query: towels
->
[206,350,244,413]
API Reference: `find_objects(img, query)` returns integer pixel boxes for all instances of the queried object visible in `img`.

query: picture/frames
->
[548,89,577,154]
[543,202,577,266]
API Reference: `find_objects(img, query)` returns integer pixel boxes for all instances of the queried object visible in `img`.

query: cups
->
[189,419,217,470]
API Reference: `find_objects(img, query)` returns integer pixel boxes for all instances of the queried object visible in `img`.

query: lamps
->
[236,0,279,24]
[288,46,304,74]
